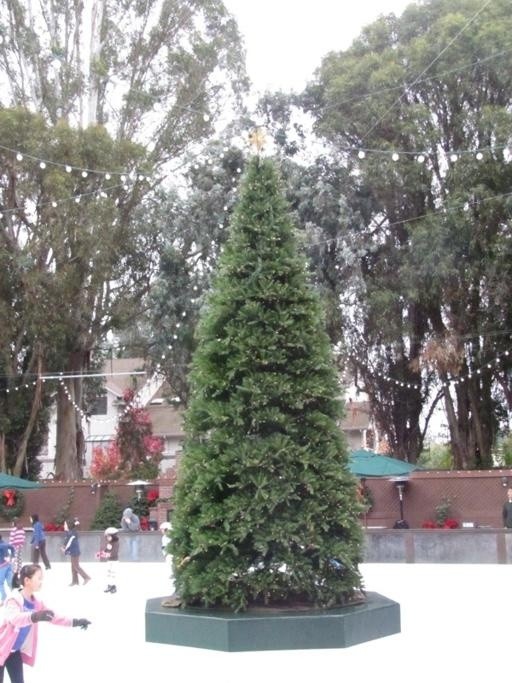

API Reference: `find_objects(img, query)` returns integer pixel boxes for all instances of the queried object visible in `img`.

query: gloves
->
[73,618,91,630]
[31,610,54,622]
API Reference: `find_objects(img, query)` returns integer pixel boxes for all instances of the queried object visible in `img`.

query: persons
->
[501,486,512,563]
[355,475,370,528]
[28,511,51,570]
[60,515,92,587]
[0,563,92,682]
[94,526,119,593]
[8,514,27,575]
[1,533,18,605]
[121,505,143,561]
[160,521,175,579]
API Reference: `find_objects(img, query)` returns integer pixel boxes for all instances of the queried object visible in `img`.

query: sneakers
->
[69,577,90,586]
[104,584,116,593]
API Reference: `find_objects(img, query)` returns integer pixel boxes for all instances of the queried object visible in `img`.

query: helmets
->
[103,527,119,535]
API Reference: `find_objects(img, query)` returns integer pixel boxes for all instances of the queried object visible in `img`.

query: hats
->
[65,520,80,530]
[159,522,171,530]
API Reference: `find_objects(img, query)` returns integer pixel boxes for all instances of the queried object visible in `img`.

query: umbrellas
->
[0,470,44,488]
[344,445,424,478]
